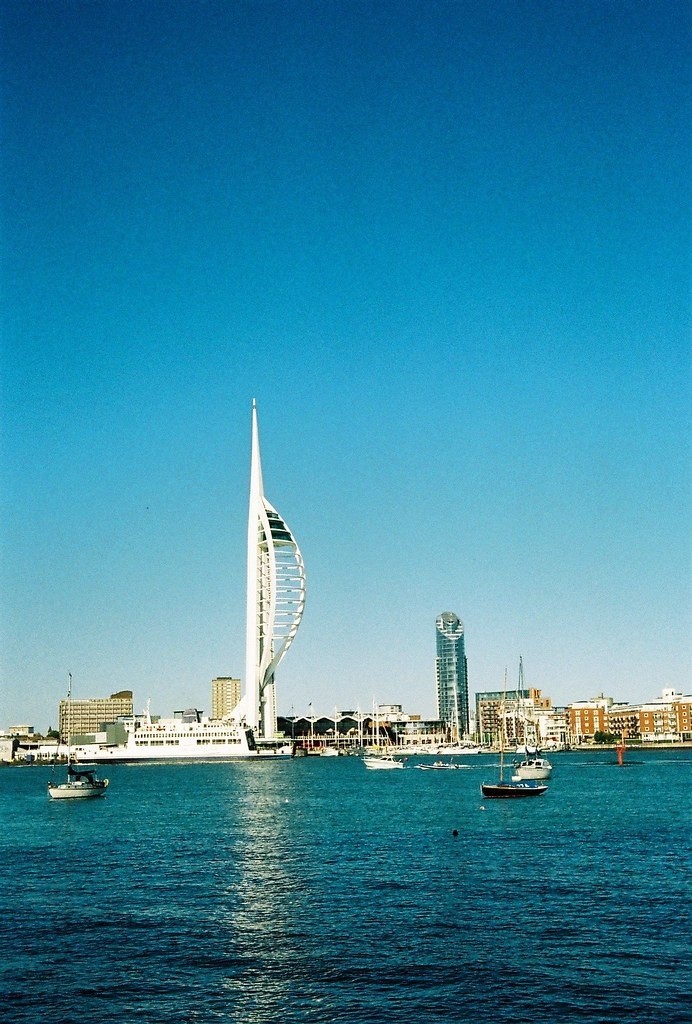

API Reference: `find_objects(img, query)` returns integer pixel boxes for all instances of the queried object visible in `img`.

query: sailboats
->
[513,656,552,780]
[479,668,548,798]
[47,672,110,799]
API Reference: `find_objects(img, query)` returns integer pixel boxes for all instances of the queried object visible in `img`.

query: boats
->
[69,698,294,765]
[362,752,403,769]
[419,763,459,769]
[294,685,576,757]
